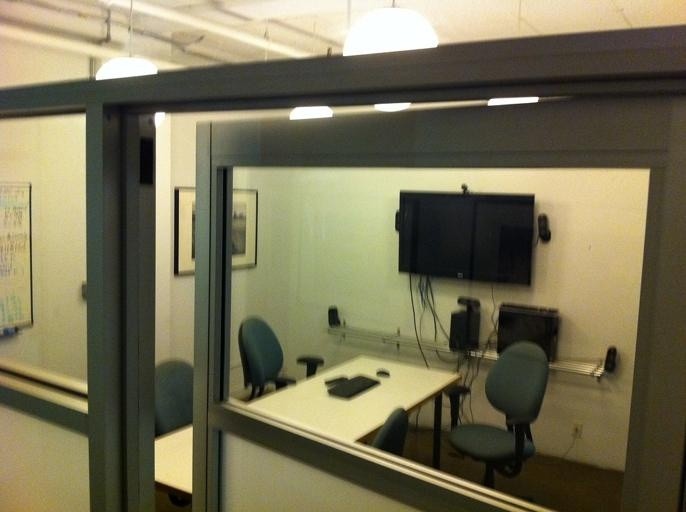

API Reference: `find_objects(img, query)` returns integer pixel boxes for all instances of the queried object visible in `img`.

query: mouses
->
[377,371,390,378]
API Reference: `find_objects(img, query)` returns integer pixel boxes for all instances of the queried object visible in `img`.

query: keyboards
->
[328,376,378,398]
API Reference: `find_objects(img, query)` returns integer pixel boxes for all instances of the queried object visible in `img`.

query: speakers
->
[328,307,341,327]
[449,310,480,351]
[604,346,616,371]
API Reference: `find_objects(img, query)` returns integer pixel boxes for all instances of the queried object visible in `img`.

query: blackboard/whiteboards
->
[0,182,33,337]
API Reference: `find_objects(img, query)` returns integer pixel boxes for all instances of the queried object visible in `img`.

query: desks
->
[153,354,462,499]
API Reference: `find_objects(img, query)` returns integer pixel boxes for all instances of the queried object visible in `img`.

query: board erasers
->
[3,326,19,335]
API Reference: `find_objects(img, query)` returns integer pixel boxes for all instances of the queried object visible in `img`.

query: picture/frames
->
[174,186,258,276]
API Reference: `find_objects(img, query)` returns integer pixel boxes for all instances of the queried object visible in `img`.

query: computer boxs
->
[497,303,559,361]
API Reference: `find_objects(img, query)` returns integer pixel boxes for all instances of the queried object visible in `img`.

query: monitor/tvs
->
[398,190,535,285]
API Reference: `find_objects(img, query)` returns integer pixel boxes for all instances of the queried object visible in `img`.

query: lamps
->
[92,1,159,81]
[341,2,438,57]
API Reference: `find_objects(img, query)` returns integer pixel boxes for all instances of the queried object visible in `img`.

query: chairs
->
[151,359,194,436]
[446,338,550,488]
[372,406,409,456]
[239,315,324,403]
